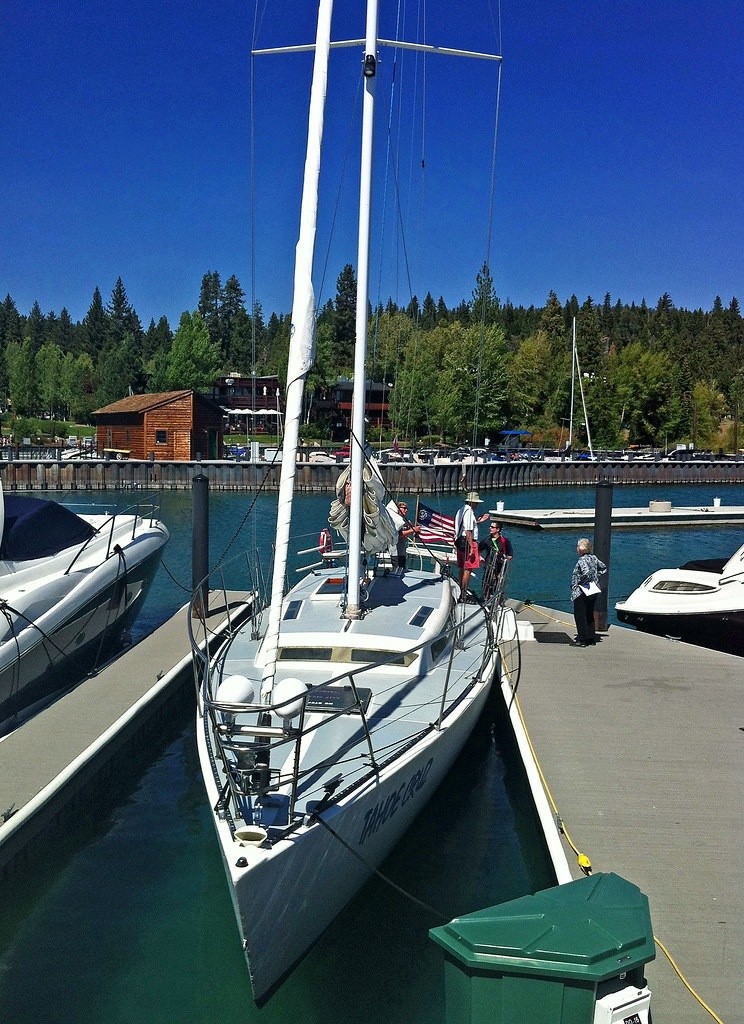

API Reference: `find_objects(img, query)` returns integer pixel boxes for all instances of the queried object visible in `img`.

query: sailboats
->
[187,0,510,1010]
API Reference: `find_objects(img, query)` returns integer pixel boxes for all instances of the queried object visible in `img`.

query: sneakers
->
[459,594,476,604]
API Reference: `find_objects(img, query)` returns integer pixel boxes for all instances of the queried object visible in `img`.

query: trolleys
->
[83,448,131,460]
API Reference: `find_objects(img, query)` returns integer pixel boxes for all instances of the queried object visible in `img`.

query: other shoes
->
[569,637,589,648]
[404,569,413,573]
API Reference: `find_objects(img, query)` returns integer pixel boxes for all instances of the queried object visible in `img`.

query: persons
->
[453,492,480,604]
[478,520,514,603]
[568,538,608,648]
[392,501,421,569]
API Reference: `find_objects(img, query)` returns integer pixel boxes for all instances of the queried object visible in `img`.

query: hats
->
[465,492,484,503]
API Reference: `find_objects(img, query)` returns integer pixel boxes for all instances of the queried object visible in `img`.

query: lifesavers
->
[320,528,332,560]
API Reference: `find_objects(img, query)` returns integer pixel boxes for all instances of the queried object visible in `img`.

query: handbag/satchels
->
[454,536,471,553]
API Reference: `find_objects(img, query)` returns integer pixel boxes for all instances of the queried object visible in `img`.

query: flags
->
[415,502,455,546]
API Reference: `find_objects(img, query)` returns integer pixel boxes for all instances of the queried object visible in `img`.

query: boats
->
[0,495,171,739]
[613,542,744,634]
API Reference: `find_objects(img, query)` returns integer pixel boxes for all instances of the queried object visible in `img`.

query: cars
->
[222,442,493,463]
[668,450,696,461]
[43,413,56,420]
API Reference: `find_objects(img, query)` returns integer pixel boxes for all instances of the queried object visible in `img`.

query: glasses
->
[399,507,408,510]
[490,526,496,528]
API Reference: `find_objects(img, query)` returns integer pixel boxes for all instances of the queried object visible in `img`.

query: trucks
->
[69,436,77,445]
[83,437,94,445]
